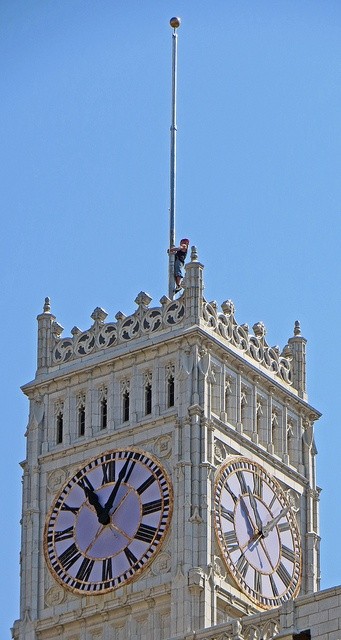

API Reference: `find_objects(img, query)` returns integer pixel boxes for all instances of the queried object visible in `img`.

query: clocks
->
[213,457,304,611]
[43,447,174,596]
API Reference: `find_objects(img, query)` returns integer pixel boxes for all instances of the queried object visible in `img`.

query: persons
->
[166,238,190,294]
